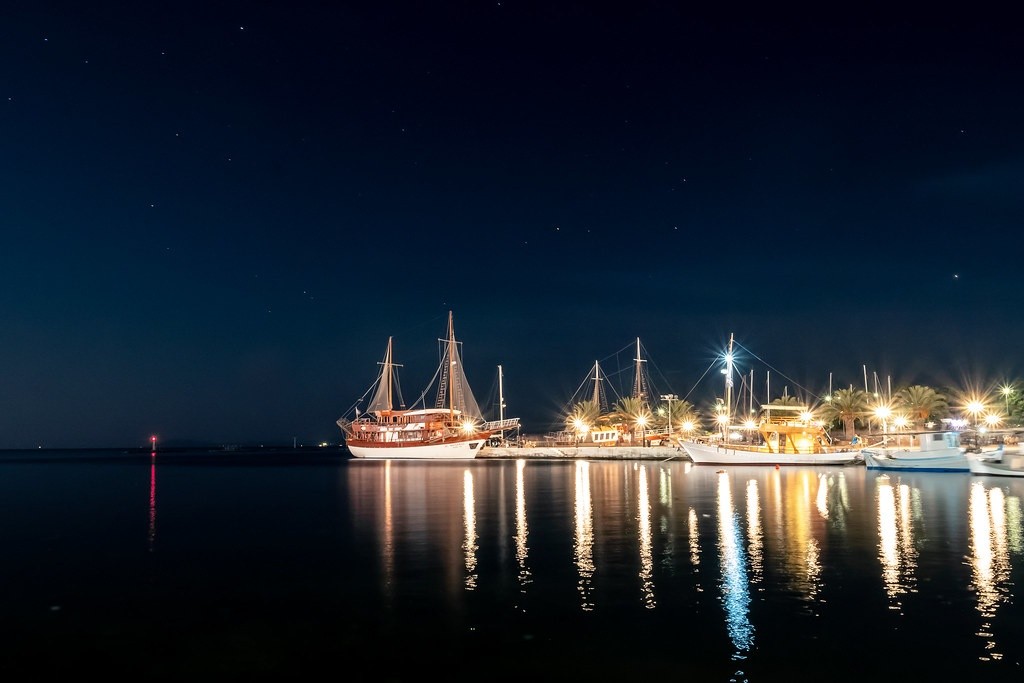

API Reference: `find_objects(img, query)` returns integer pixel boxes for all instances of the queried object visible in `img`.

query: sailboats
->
[677,331,865,464]
[336,310,491,457]
[470,338,689,463]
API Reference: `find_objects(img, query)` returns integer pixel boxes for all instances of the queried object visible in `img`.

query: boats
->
[862,431,971,472]
[965,441,1024,477]
